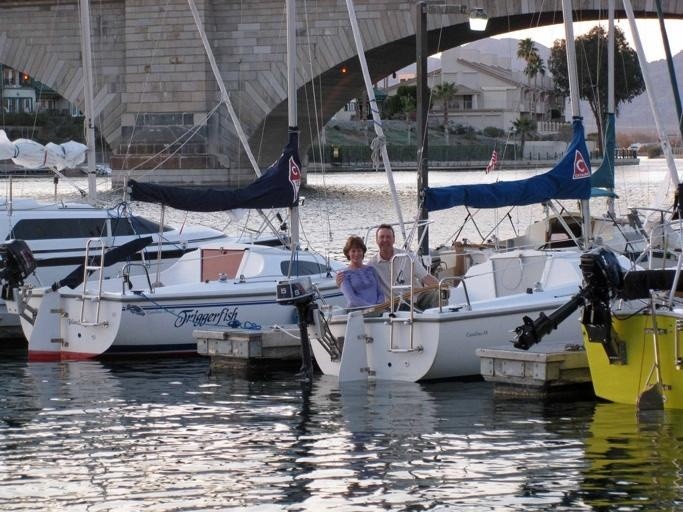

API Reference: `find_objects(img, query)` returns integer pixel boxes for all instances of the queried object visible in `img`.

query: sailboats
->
[287,0,656,389]
[0,1,292,343]
[555,0,682,412]
[23,1,361,364]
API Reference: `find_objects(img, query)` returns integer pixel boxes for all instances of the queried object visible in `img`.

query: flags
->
[484,140,496,174]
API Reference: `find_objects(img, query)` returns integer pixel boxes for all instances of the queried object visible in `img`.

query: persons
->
[335,224,448,313]
[339,234,405,318]
